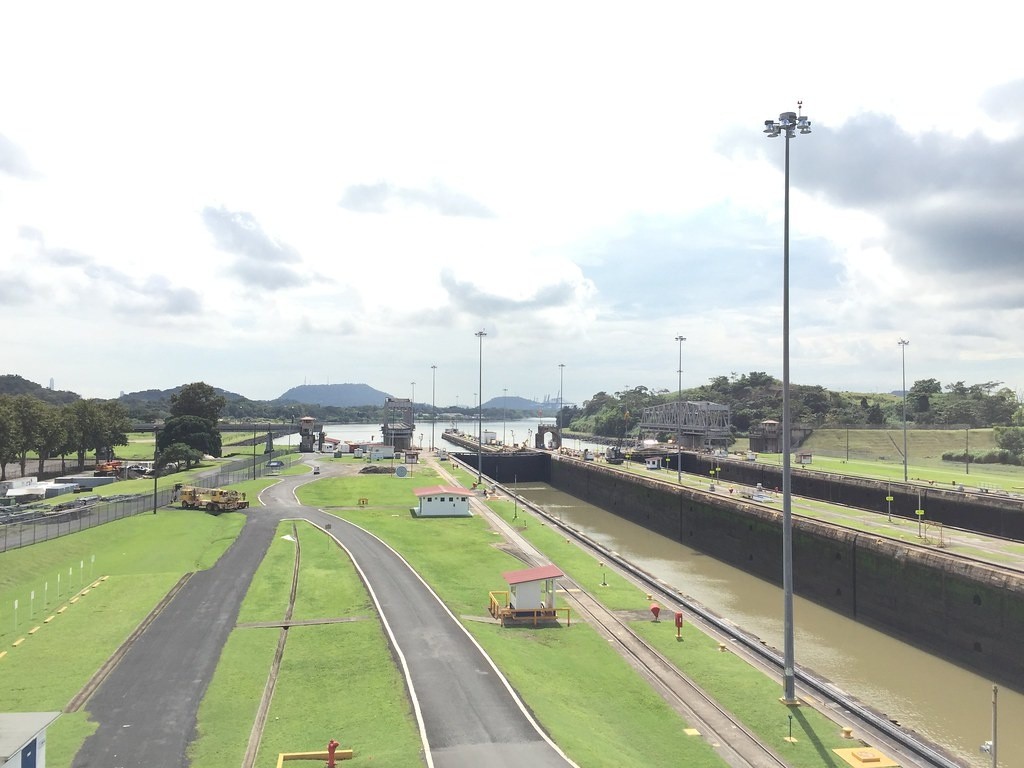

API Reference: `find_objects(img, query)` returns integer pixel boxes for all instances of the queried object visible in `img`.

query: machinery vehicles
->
[165,482,250,516]
[93,461,123,476]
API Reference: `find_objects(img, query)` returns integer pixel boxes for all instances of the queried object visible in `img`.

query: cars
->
[127,461,178,475]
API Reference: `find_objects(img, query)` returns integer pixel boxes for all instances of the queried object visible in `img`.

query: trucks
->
[604,447,625,465]
[584,450,594,461]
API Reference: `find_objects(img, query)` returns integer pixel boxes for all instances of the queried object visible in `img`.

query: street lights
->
[251,423,257,479]
[558,363,567,454]
[410,382,417,450]
[475,331,483,483]
[896,338,910,483]
[762,99,813,706]
[152,425,160,515]
[502,388,508,444]
[966,427,969,475]
[430,365,437,451]
[473,393,477,438]
[846,423,851,461]
[456,396,459,429]
[675,335,688,544]
